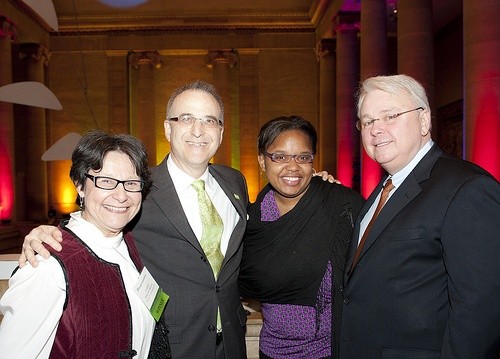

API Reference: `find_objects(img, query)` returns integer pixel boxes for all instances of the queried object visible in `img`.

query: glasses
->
[356,107,424,131]
[84,172,147,192]
[264,150,315,164]
[168,115,223,127]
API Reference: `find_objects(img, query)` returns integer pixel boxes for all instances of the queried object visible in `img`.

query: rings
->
[23,247,34,253]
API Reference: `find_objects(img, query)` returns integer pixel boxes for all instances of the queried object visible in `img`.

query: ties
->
[190,179,226,330]
[349,179,397,276]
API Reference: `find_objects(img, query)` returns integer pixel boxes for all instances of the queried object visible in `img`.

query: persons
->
[19,81,342,359]
[337,75,500,359]
[237,115,367,359]
[0,130,171,359]
